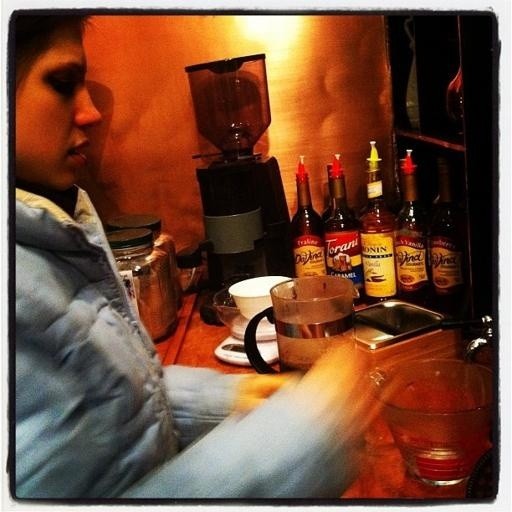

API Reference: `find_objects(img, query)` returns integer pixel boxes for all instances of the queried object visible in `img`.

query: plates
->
[228,316,279,341]
[210,335,282,363]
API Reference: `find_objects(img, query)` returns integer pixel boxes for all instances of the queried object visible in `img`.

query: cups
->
[370,357,498,490]
[225,274,289,323]
[242,275,357,381]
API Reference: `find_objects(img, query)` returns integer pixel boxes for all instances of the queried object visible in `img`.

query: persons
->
[13,11,402,498]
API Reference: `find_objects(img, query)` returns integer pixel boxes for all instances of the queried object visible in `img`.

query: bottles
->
[289,155,364,300]
[446,62,463,133]
[358,141,399,302]
[405,33,419,128]
[104,212,185,347]
[393,150,469,299]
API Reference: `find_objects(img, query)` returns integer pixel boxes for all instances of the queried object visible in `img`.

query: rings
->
[368,364,389,385]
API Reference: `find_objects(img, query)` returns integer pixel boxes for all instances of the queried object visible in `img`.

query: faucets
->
[463,315,495,361]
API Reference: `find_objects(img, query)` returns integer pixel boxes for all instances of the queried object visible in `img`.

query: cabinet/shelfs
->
[382,12,496,323]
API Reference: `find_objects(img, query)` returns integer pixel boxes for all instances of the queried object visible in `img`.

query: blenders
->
[177,53,296,300]
